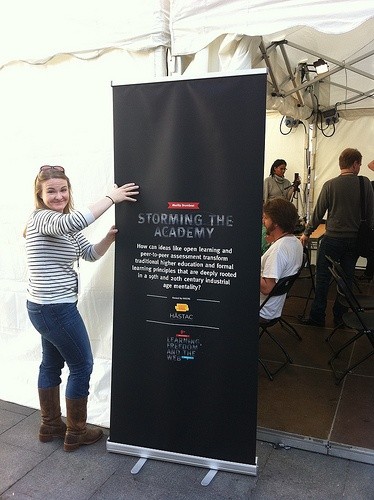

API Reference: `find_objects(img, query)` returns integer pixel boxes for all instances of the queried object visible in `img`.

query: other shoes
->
[335,322,346,328]
[299,317,324,328]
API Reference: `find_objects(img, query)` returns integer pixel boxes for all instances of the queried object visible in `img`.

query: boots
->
[39,386,67,443]
[64,395,103,453]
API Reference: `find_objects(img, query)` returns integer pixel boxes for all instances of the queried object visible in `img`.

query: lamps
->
[302,57,328,74]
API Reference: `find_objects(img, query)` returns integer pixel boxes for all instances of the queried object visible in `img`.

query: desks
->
[309,223,328,288]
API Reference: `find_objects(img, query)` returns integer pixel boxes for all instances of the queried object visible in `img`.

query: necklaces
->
[340,172,355,176]
[270,234,288,245]
[274,177,284,194]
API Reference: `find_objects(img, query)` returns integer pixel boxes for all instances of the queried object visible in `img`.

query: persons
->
[299,148,374,326]
[263,159,301,217]
[22,165,140,452]
[258,197,305,328]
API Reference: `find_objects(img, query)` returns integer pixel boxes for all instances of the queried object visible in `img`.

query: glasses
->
[40,166,65,174]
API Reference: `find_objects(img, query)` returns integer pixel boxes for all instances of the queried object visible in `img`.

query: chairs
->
[260,240,374,384]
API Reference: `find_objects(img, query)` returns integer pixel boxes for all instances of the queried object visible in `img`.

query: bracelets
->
[106,196,115,204]
